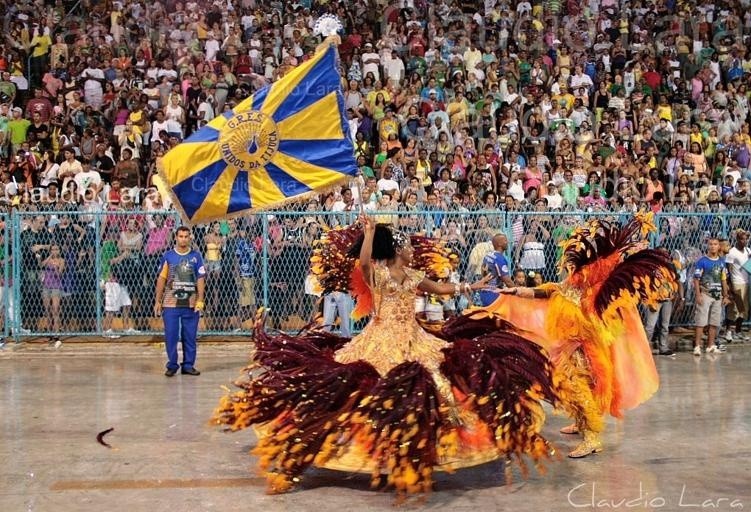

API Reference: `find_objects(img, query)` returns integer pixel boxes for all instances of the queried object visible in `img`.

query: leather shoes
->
[165,369,176,376]
[560,423,581,434]
[182,367,200,375]
[568,432,603,457]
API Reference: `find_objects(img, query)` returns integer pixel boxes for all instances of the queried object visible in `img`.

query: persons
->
[332,209,496,489]
[1,1,749,362]
[151,225,206,378]
[489,235,652,462]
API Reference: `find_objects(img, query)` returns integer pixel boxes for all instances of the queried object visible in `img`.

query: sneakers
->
[121,328,141,336]
[102,328,120,339]
[706,345,719,353]
[659,350,673,355]
[734,332,749,340]
[694,346,701,355]
[725,330,732,341]
